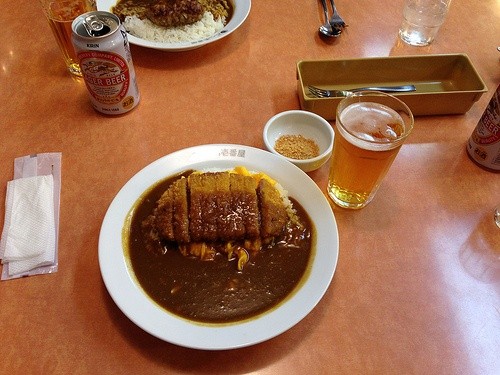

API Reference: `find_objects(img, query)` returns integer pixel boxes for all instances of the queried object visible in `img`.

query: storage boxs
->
[297,54,487,121]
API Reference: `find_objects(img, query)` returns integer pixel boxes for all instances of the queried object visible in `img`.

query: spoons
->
[319,0,342,38]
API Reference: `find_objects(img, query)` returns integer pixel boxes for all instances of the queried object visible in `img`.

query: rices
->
[120,11,228,44]
[194,168,297,220]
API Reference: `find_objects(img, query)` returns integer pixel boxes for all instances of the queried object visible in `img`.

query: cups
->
[494,204,500,229]
[399,0,451,47]
[37,0,97,78]
[327,91,415,210]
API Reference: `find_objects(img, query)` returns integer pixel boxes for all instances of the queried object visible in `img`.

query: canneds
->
[70,11,140,115]
[466,83,500,174]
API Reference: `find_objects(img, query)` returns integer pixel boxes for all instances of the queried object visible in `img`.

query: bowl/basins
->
[262,110,335,173]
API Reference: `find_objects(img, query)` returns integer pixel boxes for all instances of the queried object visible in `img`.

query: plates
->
[95,0,252,52]
[97,144,339,351]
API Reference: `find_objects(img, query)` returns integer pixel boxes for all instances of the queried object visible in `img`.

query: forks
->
[307,84,416,97]
[329,0,349,29]
